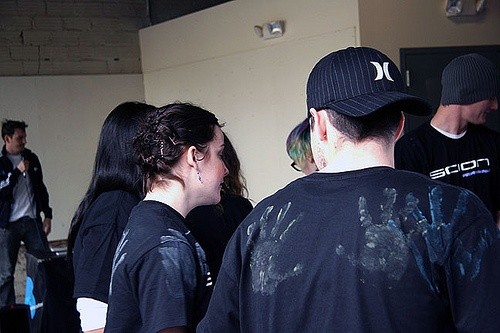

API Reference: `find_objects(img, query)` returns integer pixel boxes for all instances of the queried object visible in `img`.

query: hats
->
[440,53,499,105]
[306,47,434,119]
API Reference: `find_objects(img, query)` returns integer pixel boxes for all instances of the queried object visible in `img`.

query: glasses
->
[291,161,302,171]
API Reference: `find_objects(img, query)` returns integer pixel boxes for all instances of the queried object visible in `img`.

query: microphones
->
[21,153,26,178]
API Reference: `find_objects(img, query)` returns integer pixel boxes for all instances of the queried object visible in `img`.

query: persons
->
[186,127,254,284]
[286,117,320,176]
[68,100,158,333]
[0,118,52,308]
[394,52,499,232]
[195,45,500,333]
[104,104,229,333]
